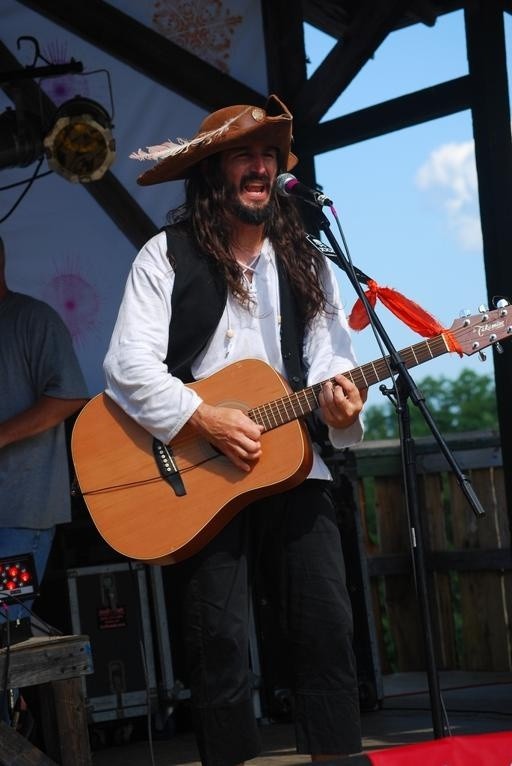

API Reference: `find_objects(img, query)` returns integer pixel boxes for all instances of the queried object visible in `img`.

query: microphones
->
[276,173,334,208]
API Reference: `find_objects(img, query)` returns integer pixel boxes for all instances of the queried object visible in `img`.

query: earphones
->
[226,326,233,338]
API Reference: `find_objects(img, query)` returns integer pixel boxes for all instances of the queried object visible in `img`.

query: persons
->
[1,234,93,606]
[101,101,376,764]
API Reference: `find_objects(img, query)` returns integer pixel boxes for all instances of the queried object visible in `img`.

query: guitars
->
[71,295,511,565]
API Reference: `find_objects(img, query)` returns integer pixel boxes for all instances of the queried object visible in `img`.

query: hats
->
[127,94,302,189]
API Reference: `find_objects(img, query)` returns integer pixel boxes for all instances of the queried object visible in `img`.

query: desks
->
[1,616,96,766]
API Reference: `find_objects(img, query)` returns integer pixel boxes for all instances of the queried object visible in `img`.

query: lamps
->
[0,57,116,184]
